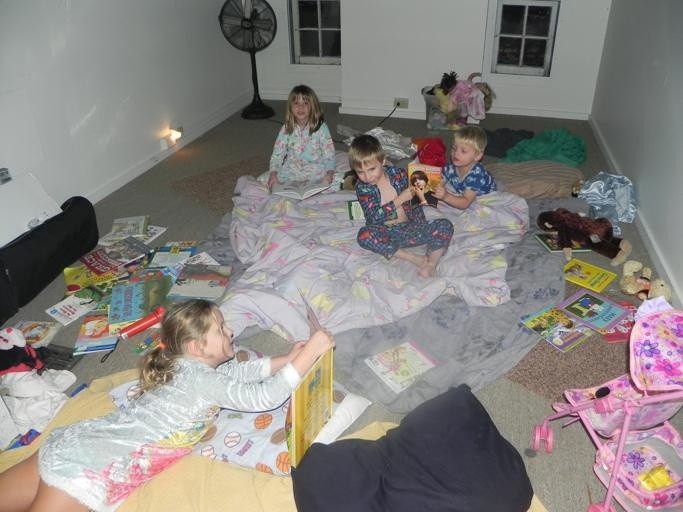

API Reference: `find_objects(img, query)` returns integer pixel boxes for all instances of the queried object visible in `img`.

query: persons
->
[410,170,438,207]
[430,126,496,211]
[348,134,453,279]
[257,84,336,189]
[0,297,336,512]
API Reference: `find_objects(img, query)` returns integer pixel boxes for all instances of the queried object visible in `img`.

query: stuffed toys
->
[622,260,674,302]
[537,208,633,265]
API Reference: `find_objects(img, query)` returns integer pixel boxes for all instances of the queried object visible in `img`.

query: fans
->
[217,0,279,119]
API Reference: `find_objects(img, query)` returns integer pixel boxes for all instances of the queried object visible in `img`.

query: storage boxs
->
[421,85,475,131]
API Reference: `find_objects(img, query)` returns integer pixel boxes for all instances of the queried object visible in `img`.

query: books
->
[520,227,639,354]
[291,284,334,469]
[366,340,438,395]
[407,156,442,209]
[16,213,232,352]
[271,181,341,200]
